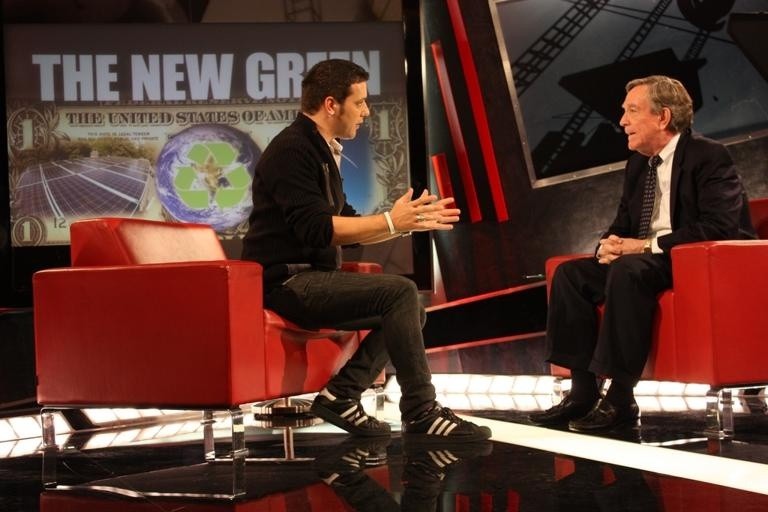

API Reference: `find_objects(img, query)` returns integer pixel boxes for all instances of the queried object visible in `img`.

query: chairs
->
[545,198,768,442]
[33,218,386,504]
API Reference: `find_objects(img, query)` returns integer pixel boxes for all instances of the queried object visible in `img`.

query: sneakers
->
[400,400,491,443]
[308,385,391,439]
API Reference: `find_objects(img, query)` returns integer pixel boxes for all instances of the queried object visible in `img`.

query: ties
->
[639,156,662,240]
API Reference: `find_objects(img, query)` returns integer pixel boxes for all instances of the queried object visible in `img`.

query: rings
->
[416,213,426,223]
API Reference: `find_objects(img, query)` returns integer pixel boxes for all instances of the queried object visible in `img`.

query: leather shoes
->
[525,391,604,430]
[569,397,644,434]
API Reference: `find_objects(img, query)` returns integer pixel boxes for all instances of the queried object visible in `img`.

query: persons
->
[240,58,492,444]
[541,425,666,512]
[309,434,493,512]
[525,74,758,432]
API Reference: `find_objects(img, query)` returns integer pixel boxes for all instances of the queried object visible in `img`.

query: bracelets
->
[400,231,411,238]
[384,210,396,237]
[643,238,652,254]
